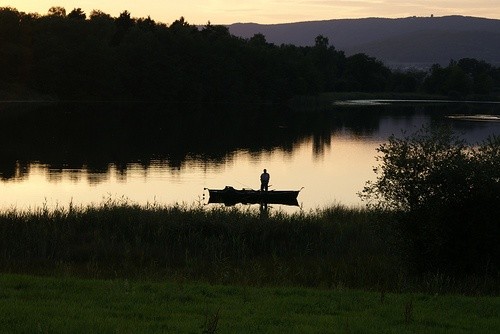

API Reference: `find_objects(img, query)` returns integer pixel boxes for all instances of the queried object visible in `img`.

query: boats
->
[208,188,299,198]
[208,198,297,206]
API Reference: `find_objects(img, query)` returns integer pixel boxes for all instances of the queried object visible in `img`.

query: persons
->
[260,168,270,192]
